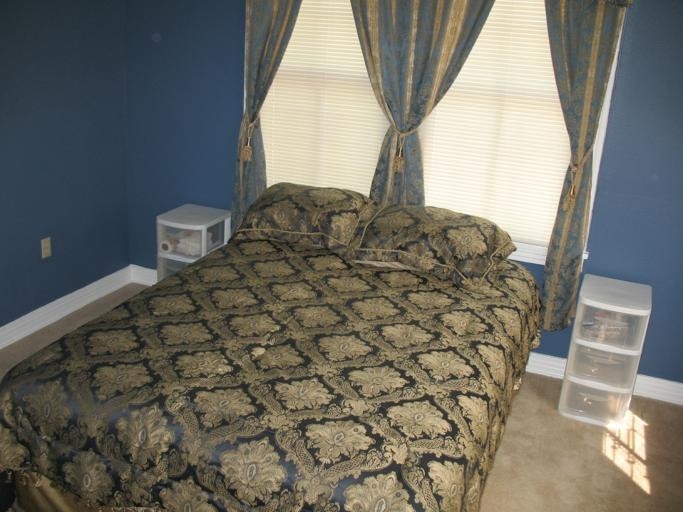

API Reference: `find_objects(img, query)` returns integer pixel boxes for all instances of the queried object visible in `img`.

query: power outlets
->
[41,237,51,259]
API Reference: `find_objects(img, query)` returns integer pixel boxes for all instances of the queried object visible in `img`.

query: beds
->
[0,243,541,512]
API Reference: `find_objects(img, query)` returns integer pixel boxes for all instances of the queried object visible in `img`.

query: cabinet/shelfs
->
[156,203,231,282]
[557,274,652,429]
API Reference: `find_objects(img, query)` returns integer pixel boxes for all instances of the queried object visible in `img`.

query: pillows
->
[357,204,515,289]
[235,181,370,250]
